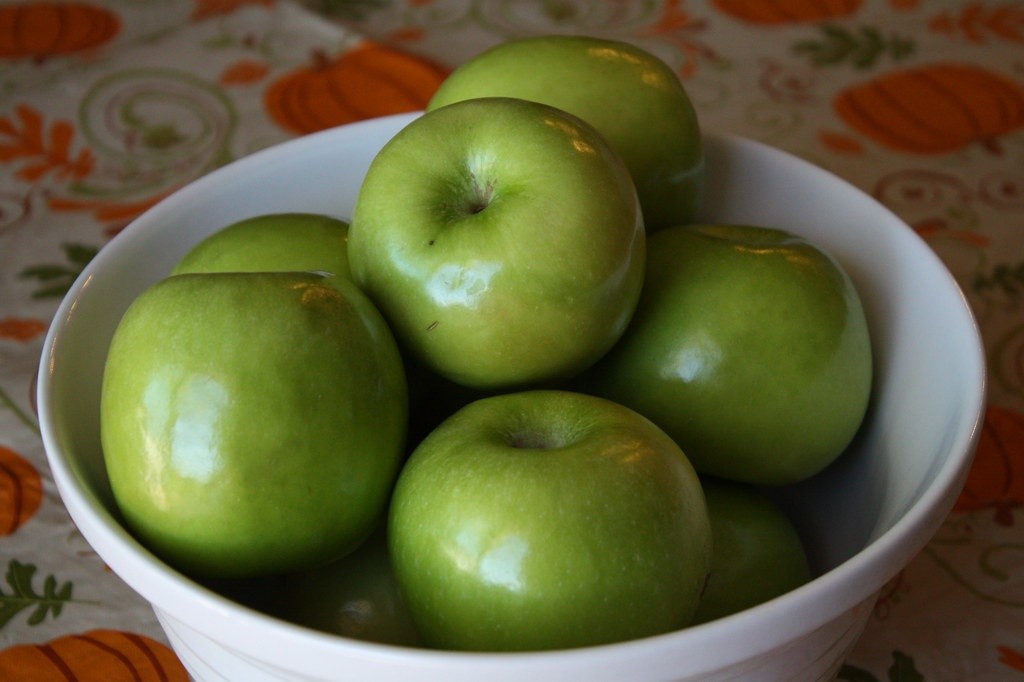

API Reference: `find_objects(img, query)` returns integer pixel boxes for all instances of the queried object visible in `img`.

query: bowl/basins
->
[31,106,988,682]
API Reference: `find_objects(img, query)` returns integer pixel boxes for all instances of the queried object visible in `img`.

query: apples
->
[96,35,877,654]
